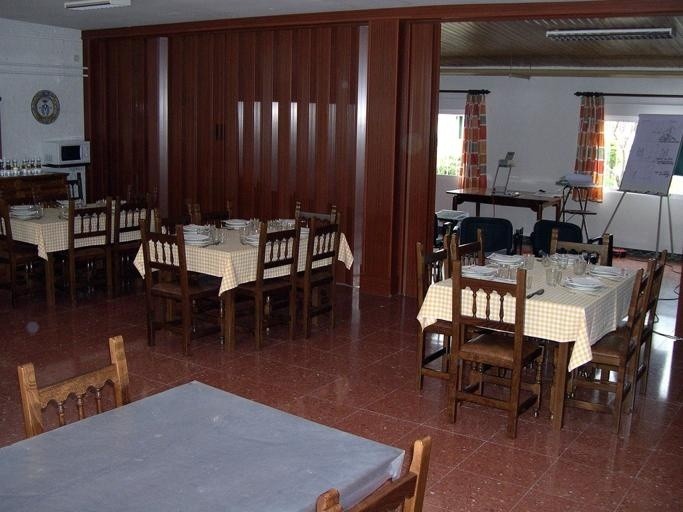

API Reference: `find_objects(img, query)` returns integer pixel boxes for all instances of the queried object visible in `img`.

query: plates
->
[181,218,312,247]
[456,251,627,293]
[8,200,128,219]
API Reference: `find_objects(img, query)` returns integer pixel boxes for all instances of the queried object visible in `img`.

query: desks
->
[445,188,566,222]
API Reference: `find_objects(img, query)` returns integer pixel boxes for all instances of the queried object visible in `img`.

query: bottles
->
[0,157,42,178]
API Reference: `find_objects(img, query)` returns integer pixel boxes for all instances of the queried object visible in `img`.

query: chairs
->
[2,173,155,313]
[133,201,353,356]
[316,435,432,512]
[416,217,667,439]
[18,334,131,439]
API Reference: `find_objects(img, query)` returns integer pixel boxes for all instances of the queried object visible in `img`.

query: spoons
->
[526,288,545,300]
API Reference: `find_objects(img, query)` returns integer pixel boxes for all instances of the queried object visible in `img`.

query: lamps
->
[62,0,130,13]
[542,26,675,44]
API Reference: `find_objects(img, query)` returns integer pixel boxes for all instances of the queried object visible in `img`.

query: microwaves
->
[42,141,92,166]
